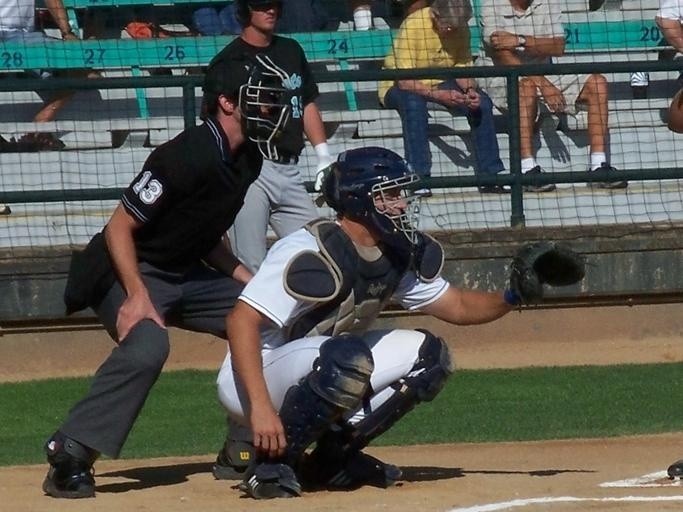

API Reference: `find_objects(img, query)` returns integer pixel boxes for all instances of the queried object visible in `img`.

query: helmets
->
[322,145,422,246]
[244,54,311,164]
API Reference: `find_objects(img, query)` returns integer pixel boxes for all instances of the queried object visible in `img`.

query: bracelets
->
[516,34,527,48]
[462,86,475,94]
[61,31,74,38]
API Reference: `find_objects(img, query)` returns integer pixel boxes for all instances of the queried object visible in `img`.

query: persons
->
[0,1,82,149]
[472,1,628,195]
[40,54,305,499]
[190,2,246,76]
[666,73,683,133]
[656,2,683,55]
[213,149,590,500]
[197,1,334,281]
[376,1,513,195]
[333,1,429,68]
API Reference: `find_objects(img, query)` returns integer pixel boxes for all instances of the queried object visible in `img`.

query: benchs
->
[0,1,683,250]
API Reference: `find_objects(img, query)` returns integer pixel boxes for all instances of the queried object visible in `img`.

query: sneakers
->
[240,469,295,499]
[210,440,249,482]
[478,170,513,193]
[304,451,367,488]
[18,130,69,150]
[587,162,629,190]
[38,453,96,499]
[415,186,432,196]
[521,164,557,193]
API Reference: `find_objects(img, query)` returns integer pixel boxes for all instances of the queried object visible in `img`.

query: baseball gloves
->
[510,242,584,305]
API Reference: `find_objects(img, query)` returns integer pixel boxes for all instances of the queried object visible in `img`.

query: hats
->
[201,57,253,96]
